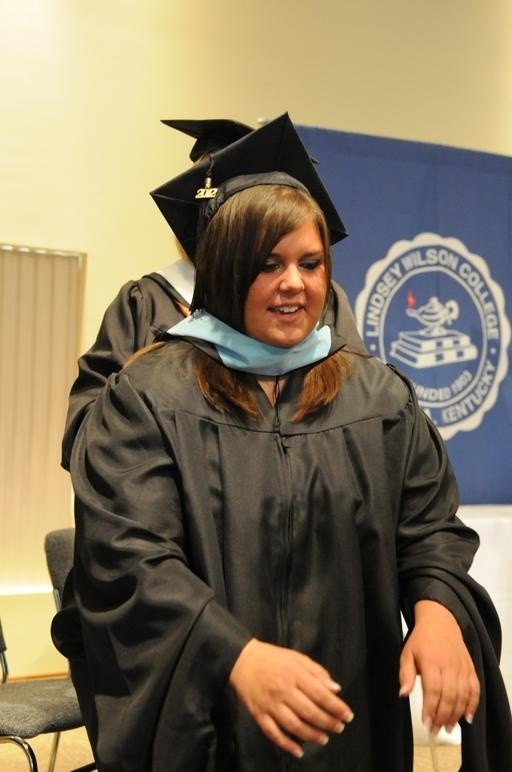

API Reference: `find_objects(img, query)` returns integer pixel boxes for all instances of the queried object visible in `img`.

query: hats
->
[149,112,349,272]
[160,120,320,164]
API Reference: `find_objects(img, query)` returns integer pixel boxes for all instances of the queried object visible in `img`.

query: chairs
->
[44,526,75,613]
[0,621,97,772]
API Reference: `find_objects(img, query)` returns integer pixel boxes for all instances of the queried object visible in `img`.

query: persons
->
[49,115,511,771]
[59,119,260,470]
